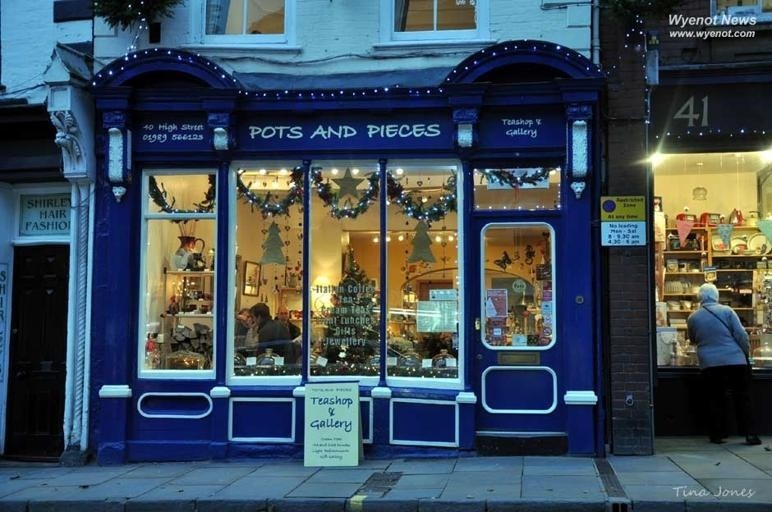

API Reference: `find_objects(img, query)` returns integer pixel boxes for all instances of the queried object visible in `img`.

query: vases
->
[190,251,205,271]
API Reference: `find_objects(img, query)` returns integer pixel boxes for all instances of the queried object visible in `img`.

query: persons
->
[684,283,763,445]
[273,305,301,338]
[251,302,297,365]
[235,308,262,353]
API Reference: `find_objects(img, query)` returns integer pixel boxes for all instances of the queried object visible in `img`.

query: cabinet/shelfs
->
[655,224,771,361]
[157,270,217,369]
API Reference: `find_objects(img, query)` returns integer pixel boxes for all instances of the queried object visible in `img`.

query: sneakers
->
[709,433,762,447]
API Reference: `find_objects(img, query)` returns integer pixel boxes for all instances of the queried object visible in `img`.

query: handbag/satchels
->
[742,346,751,371]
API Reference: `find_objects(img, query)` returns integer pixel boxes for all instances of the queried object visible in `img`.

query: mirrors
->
[242,259,261,298]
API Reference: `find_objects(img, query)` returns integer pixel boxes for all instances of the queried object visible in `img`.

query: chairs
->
[234,339,302,365]
